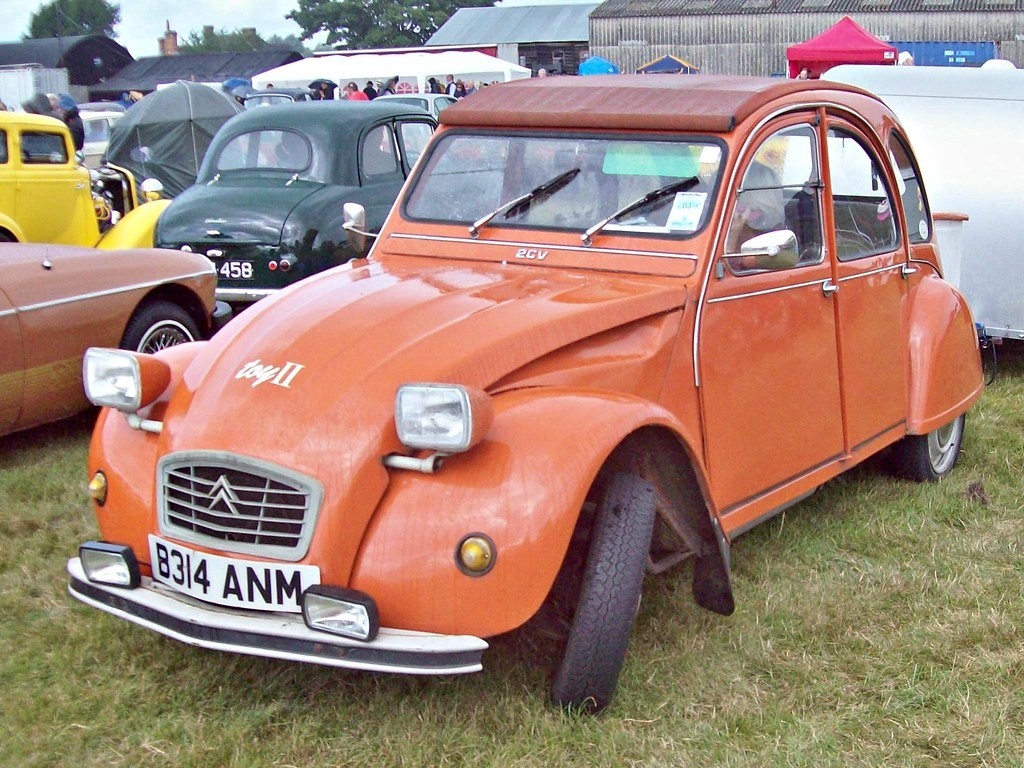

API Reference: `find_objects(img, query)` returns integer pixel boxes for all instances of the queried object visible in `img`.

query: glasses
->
[738,199,786,232]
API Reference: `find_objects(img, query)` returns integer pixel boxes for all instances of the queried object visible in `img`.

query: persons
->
[538,68,546,77]
[0,92,85,156]
[260,83,274,104]
[237,97,246,105]
[383,81,396,95]
[707,160,786,271]
[343,81,378,100]
[898,51,914,66]
[119,89,142,109]
[785,170,820,247]
[318,83,334,100]
[796,68,808,80]
[428,74,477,100]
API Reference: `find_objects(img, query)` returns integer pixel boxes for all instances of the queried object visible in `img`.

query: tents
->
[252,51,532,108]
[786,15,899,80]
[637,53,699,75]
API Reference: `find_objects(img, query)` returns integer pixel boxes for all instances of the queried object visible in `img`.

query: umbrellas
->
[223,77,250,88]
[308,79,338,89]
[230,86,258,99]
[379,75,399,96]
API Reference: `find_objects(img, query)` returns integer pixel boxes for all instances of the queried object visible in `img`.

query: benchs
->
[778,199,893,259]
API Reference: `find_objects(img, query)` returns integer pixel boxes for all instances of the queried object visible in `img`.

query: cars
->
[0,110,173,253]
[68,76,986,713]
[72,88,463,304]
[778,59,1024,342]
[0,240,232,440]
